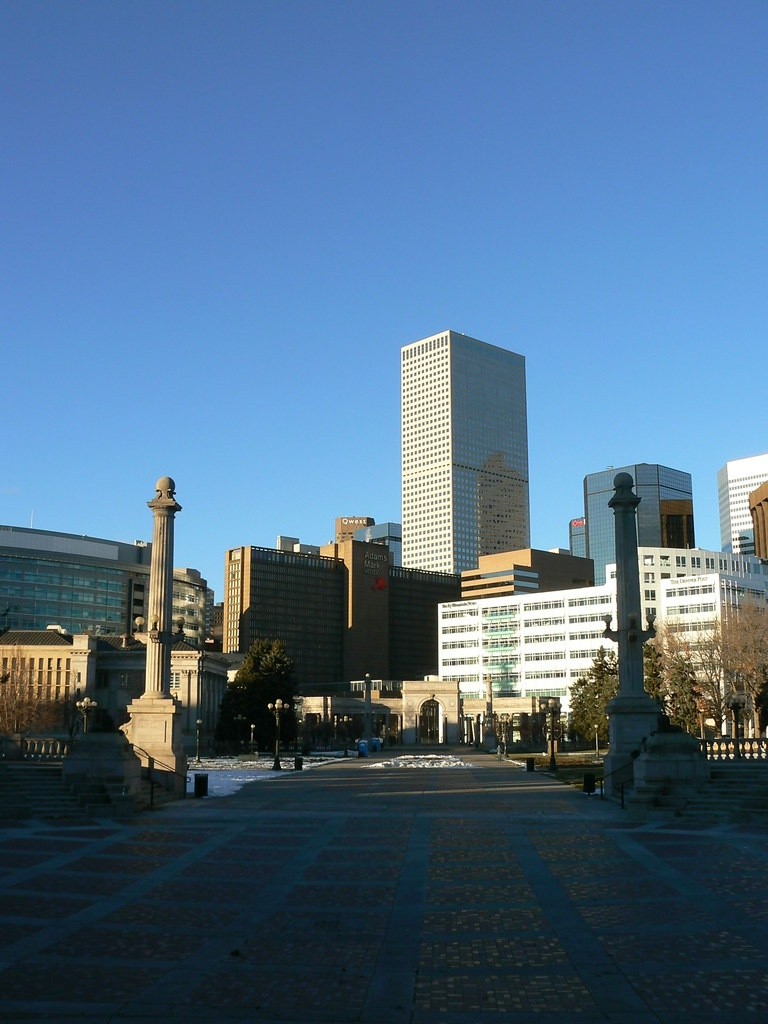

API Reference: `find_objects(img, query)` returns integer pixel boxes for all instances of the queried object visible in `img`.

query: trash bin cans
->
[371,739,380,752]
[295,752,303,770]
[359,740,368,757]
[195,773,208,799]
[526,757,535,772]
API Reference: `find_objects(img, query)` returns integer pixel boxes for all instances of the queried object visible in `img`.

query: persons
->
[496,745,503,764]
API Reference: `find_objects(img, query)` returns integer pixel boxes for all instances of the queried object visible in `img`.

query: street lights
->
[250,724,255,754]
[340,715,353,758]
[75,697,98,732]
[725,693,747,758]
[540,699,562,772]
[496,714,513,757]
[195,719,202,762]
[594,725,600,757]
[268,698,290,771]
